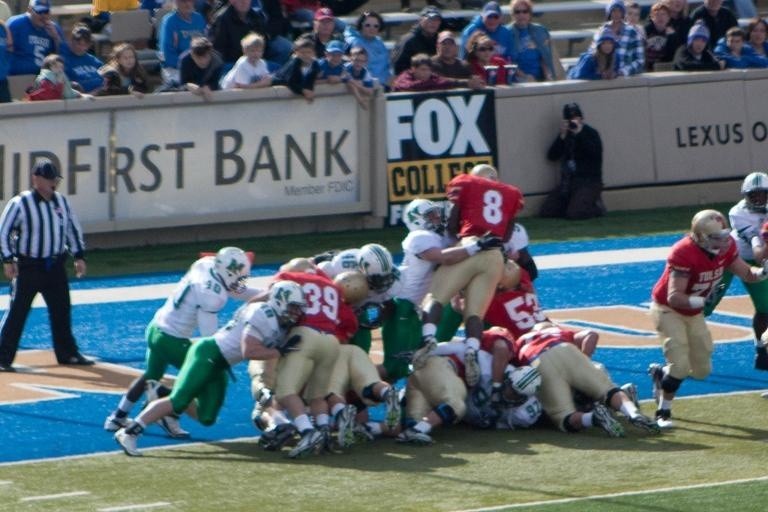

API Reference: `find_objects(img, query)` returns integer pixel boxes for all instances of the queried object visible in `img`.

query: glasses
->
[474,44,494,52]
[514,9,533,15]
[363,22,381,29]
[31,7,50,16]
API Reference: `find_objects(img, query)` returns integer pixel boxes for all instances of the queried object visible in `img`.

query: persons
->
[242,270,370,449]
[450,258,642,407]
[217,32,272,89]
[690,0,742,46]
[506,0,566,84]
[344,343,544,456]
[341,46,378,111]
[210,1,280,79]
[394,327,519,445]
[412,164,523,386]
[282,0,347,33]
[6,0,65,100]
[642,4,681,72]
[90,1,151,49]
[115,281,303,458]
[713,26,768,69]
[254,0,293,30]
[389,53,453,93]
[372,197,504,384]
[178,37,226,102]
[0,21,13,103]
[344,11,392,91]
[1,159,97,373]
[22,55,80,99]
[99,43,155,101]
[722,0,760,40]
[430,30,485,87]
[540,104,608,219]
[318,343,399,444]
[430,223,541,346]
[723,171,768,372]
[101,246,266,438]
[623,0,646,44]
[673,20,720,71]
[324,245,402,357]
[57,22,105,97]
[389,5,442,75]
[466,30,534,87]
[530,6,565,80]
[745,18,768,68]
[316,39,348,86]
[567,28,620,80]
[247,257,360,458]
[588,2,647,76]
[272,35,321,101]
[664,0,692,43]
[650,210,768,429]
[460,1,517,81]
[302,7,345,55]
[154,0,207,89]
[512,322,661,443]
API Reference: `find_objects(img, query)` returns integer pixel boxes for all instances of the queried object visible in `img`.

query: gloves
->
[465,231,504,255]
[489,384,505,412]
[703,282,728,309]
[273,334,302,357]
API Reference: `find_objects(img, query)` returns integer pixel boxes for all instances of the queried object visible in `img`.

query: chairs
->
[6,74,39,103]
[108,8,160,74]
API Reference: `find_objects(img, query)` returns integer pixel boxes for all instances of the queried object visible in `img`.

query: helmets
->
[357,243,396,287]
[509,364,544,396]
[401,196,445,233]
[531,321,553,332]
[741,170,768,195]
[333,269,368,305]
[279,258,315,274]
[215,245,249,287]
[269,281,307,318]
[472,163,499,180]
[690,208,728,253]
[499,259,520,289]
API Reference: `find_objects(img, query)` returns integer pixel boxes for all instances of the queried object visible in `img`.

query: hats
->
[34,160,64,180]
[482,2,501,19]
[422,7,442,21]
[28,0,49,11]
[564,103,580,119]
[606,0,626,21]
[438,30,455,44]
[325,38,346,55]
[314,7,334,22]
[595,25,619,48]
[687,22,711,46]
[72,26,92,41]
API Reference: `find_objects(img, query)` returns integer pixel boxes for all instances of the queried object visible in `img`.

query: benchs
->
[47,3,94,21]
[335,1,703,39]
[558,56,581,72]
[383,16,757,52]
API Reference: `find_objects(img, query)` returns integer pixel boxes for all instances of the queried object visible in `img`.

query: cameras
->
[568,121,580,130]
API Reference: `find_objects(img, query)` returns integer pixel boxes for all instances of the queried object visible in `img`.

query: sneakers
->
[655,411,676,429]
[649,363,661,405]
[632,414,661,435]
[286,428,326,459]
[337,403,359,449]
[382,383,403,434]
[463,347,481,388]
[352,421,377,444]
[259,424,296,450]
[398,428,434,444]
[146,379,159,399]
[104,415,134,430]
[412,338,440,371]
[113,427,143,459]
[158,416,190,440]
[595,401,626,438]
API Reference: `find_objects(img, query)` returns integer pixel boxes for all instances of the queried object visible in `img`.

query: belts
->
[19,252,68,268]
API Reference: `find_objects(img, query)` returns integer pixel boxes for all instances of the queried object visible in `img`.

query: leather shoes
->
[58,351,94,366]
[0,358,16,373]
